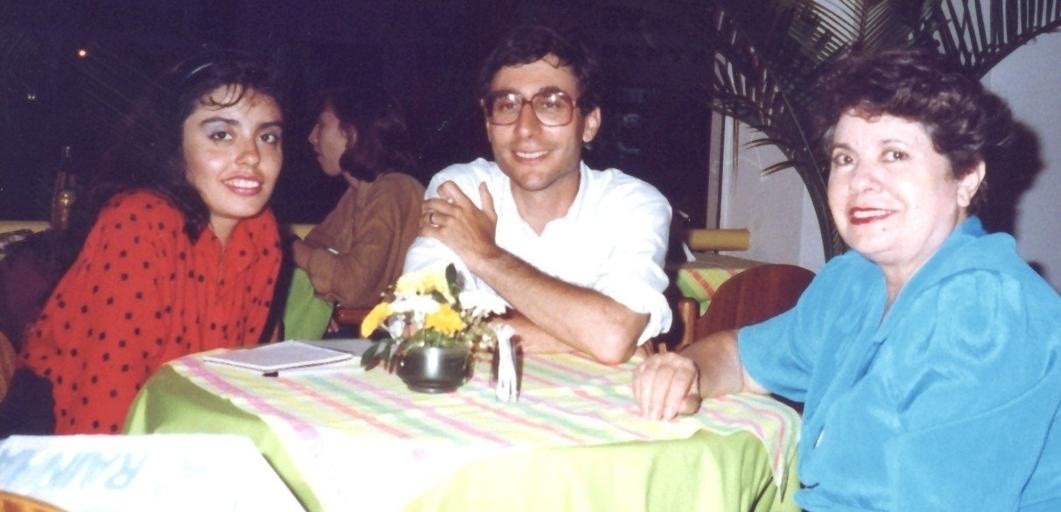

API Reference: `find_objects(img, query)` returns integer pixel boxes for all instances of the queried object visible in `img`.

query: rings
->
[427,211,435,225]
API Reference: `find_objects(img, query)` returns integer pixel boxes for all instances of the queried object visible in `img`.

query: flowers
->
[359,260,507,374]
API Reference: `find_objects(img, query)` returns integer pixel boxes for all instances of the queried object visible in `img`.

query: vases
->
[395,339,473,391]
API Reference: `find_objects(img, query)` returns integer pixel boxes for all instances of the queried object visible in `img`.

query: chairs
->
[665,249,819,346]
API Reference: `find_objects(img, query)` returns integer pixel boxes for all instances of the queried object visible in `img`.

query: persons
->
[278,82,429,336]
[1,62,294,438]
[383,28,672,364]
[634,42,1060,512]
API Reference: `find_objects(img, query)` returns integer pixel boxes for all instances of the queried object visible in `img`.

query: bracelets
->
[284,231,299,257]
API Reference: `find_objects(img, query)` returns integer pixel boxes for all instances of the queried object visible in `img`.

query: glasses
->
[482,88,579,128]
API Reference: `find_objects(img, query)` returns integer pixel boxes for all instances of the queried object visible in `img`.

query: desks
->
[674,252,767,302]
[155,332,809,510]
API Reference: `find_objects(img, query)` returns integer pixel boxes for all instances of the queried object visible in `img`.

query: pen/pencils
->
[263,365,360,378]
